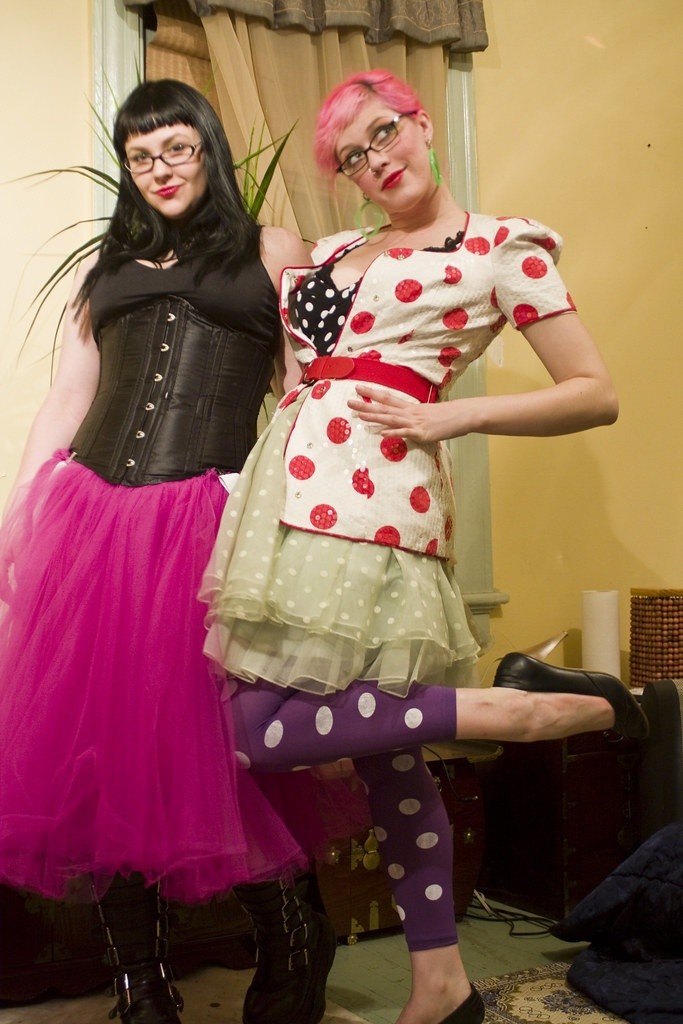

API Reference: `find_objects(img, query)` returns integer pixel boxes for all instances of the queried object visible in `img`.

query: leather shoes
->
[439,983,485,1024]
[493,652,650,743]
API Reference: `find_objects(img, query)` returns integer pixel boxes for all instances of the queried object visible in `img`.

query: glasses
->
[336,114,404,177]
[124,142,201,174]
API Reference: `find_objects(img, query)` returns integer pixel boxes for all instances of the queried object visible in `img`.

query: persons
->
[200,67,649,1024]
[0,80,336,1024]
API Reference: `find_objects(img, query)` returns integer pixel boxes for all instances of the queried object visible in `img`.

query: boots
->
[232,858,337,1024]
[90,874,184,1024]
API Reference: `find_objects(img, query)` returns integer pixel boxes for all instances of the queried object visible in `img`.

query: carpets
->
[470,958,631,1024]
[0,966,373,1024]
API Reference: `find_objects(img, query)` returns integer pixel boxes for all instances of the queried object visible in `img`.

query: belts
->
[299,357,439,404]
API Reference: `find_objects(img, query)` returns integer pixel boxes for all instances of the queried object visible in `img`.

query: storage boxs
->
[0,729,640,1007]
